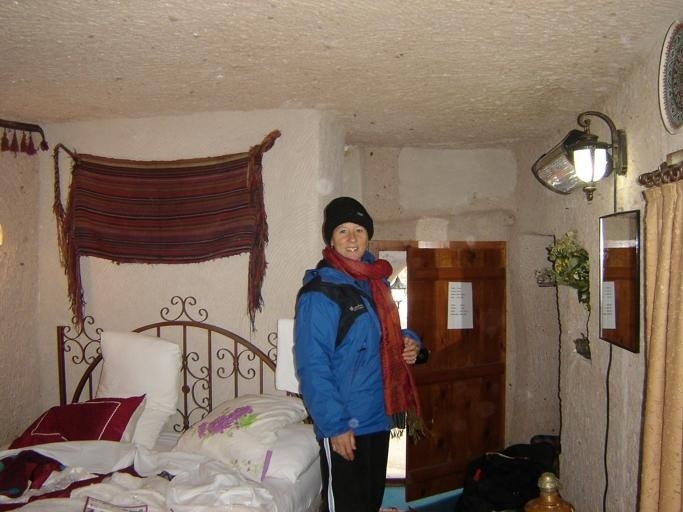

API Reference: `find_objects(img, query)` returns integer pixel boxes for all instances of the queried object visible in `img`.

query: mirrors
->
[598,210,640,354]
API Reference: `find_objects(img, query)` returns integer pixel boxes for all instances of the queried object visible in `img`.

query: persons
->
[291,197,424,511]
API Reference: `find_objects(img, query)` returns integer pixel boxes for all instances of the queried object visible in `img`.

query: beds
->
[0,296,323,512]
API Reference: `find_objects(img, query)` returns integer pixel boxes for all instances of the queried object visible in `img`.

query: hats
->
[322,197,374,247]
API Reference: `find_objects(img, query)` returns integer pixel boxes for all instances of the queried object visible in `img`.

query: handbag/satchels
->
[456,435,560,511]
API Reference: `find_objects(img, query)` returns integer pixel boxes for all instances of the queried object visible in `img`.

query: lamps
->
[570,110,627,201]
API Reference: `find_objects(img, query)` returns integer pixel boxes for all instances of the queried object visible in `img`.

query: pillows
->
[133,408,170,452]
[8,397,146,451]
[225,424,320,485]
[173,392,309,456]
[99,333,181,413]
